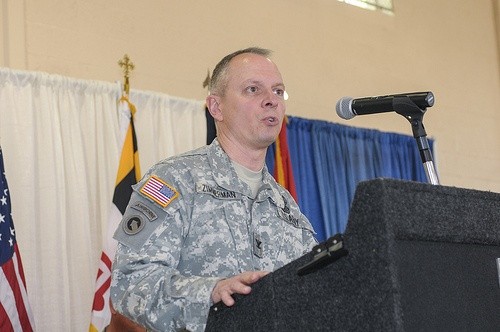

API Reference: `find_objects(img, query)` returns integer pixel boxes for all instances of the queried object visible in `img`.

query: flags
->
[87,93,141,332]
[264,112,298,204]
[0,141,36,332]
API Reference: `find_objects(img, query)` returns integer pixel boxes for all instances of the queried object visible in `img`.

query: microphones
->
[335,91,434,120]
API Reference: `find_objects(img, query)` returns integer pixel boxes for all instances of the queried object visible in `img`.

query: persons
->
[107,47,319,331]
